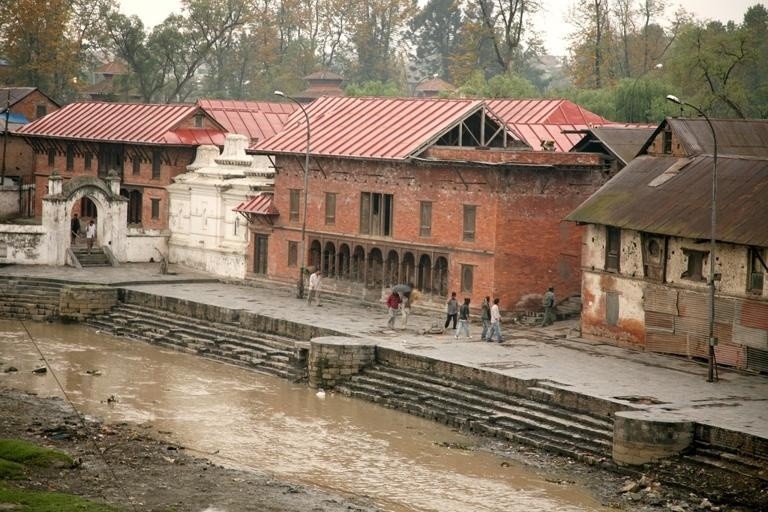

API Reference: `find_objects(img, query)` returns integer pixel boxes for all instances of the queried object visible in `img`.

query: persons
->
[71,214,80,244]
[307,268,323,306]
[539,287,556,327]
[386,292,400,331]
[480,295,491,340]
[454,298,473,341]
[444,292,459,330]
[400,282,415,331]
[85,220,96,256]
[487,298,505,344]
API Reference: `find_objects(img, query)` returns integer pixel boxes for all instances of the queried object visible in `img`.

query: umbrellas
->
[392,285,413,302]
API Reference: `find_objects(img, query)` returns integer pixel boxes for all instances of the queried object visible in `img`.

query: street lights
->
[629,63,664,123]
[665,93,718,383]
[273,90,311,301]
